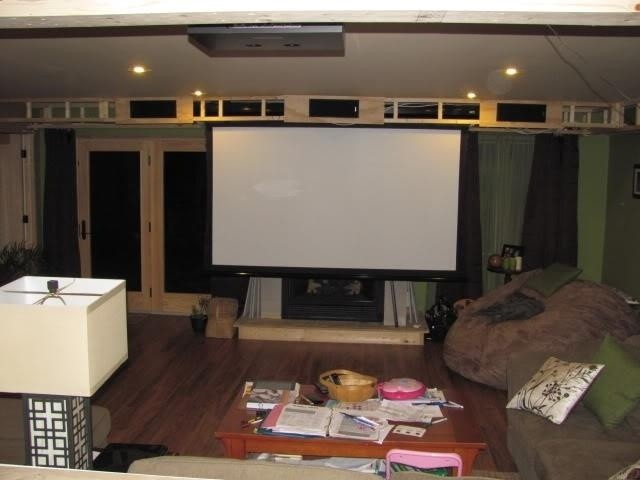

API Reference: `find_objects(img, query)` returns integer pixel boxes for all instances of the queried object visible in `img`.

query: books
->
[242,377,384,442]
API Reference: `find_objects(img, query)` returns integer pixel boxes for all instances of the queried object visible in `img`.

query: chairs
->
[383,447,462,480]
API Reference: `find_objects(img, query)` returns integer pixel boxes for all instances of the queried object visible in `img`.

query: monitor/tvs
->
[200,119,469,286]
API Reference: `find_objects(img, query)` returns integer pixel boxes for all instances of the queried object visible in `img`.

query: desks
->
[487,265,528,284]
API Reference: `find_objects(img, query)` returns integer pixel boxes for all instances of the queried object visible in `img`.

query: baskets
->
[319,369,377,402]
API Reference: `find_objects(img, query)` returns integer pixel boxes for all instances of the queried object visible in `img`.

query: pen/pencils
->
[423,417,448,428]
[242,419,264,427]
[351,414,379,429]
[247,416,261,422]
[442,400,465,409]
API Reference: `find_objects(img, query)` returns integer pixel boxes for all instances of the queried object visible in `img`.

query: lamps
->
[186,27,346,59]
[0,276,128,468]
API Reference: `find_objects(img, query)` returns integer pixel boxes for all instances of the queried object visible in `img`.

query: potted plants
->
[190,296,208,332]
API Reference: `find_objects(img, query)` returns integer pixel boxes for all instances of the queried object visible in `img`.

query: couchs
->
[1,399,498,478]
[442,263,639,392]
[507,334,640,480]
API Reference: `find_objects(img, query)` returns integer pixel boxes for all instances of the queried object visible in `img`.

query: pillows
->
[507,353,605,427]
[577,333,640,431]
[526,264,581,297]
